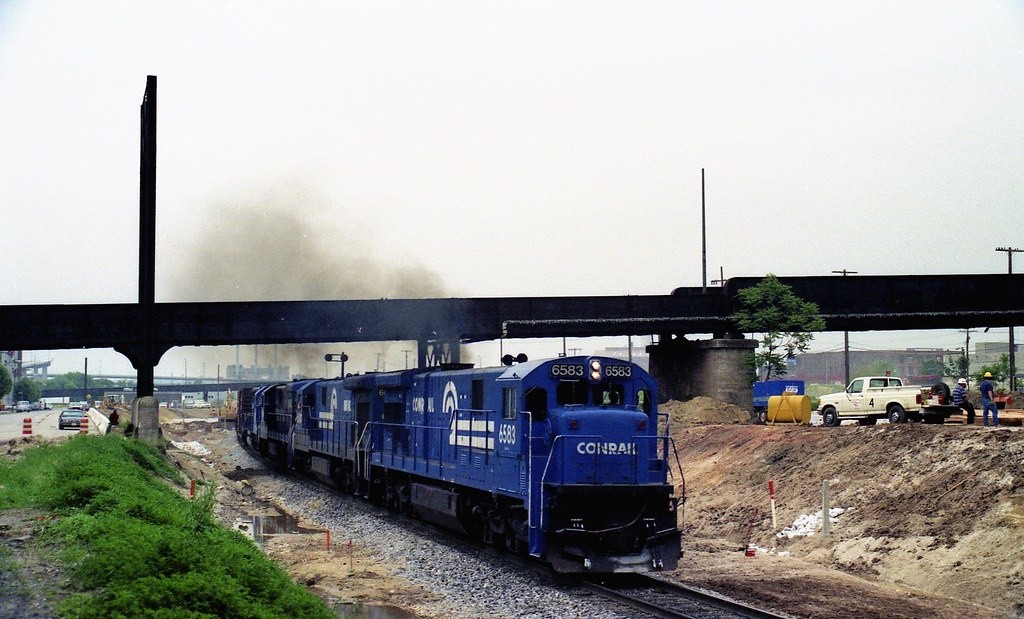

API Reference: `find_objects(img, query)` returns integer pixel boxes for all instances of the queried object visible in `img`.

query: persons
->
[109,410,119,425]
[953,378,976,424]
[979,372,999,427]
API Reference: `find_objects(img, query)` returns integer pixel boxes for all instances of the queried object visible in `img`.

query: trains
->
[236,354,689,574]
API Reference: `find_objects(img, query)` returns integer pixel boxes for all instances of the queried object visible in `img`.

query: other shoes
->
[983,424,990,427]
[995,424,1002,428]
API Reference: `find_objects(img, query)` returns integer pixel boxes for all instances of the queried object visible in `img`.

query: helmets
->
[958,377,967,384]
[983,371,992,377]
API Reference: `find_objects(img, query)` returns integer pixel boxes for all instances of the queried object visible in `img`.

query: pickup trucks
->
[817,370,964,424]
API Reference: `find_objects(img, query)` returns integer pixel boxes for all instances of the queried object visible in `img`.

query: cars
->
[57,411,88,430]
[0,400,53,414]
[158,400,211,409]
[68,401,102,411]
